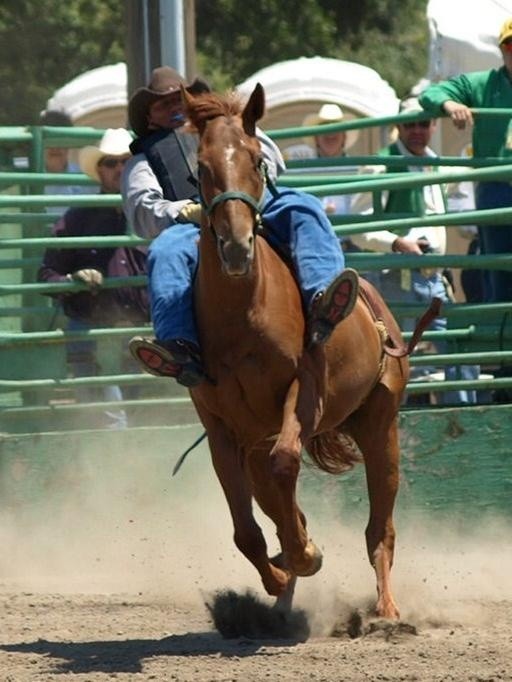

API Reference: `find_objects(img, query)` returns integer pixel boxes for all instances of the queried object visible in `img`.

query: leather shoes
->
[307,265,360,351]
[128,335,206,387]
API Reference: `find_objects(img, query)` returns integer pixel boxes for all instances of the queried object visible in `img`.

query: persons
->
[347,98,479,404]
[35,128,149,429]
[279,102,361,253]
[444,142,496,304]
[25,112,101,226]
[118,66,359,390]
[415,17,511,303]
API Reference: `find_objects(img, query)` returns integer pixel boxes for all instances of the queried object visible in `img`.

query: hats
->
[497,16,512,48]
[300,102,362,152]
[24,111,73,128]
[396,96,423,114]
[77,127,137,185]
[128,65,211,139]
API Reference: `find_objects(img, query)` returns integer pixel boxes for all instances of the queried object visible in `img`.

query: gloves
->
[68,267,103,298]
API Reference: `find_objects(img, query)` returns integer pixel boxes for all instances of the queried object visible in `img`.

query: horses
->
[190,80,410,624]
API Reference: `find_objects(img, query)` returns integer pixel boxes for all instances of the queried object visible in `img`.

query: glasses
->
[95,158,131,169]
[402,119,432,129]
